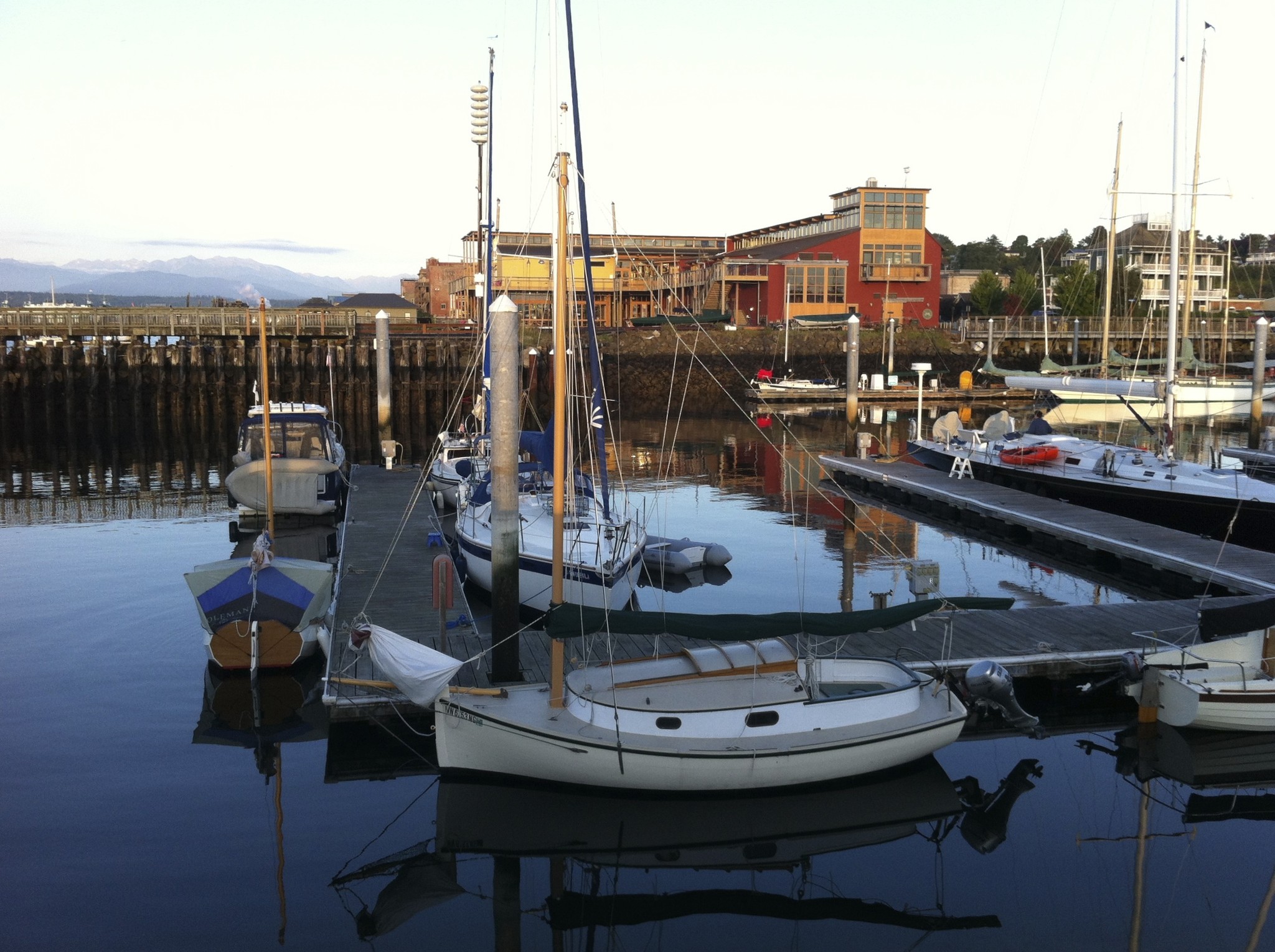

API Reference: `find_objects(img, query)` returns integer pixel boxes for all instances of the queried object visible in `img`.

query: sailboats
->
[328,753,1046,952]
[750,282,841,390]
[190,659,331,952]
[906,0,1275,514]
[180,297,337,672]
[428,0,733,626]
[322,151,1042,791]
[756,403,835,513]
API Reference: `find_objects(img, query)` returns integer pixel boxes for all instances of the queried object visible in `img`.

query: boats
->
[1075,734,1274,824]
[1073,625,1274,734]
[793,312,863,328]
[225,399,352,517]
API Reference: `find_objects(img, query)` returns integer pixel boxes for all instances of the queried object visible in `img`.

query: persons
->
[1026,410,1052,435]
